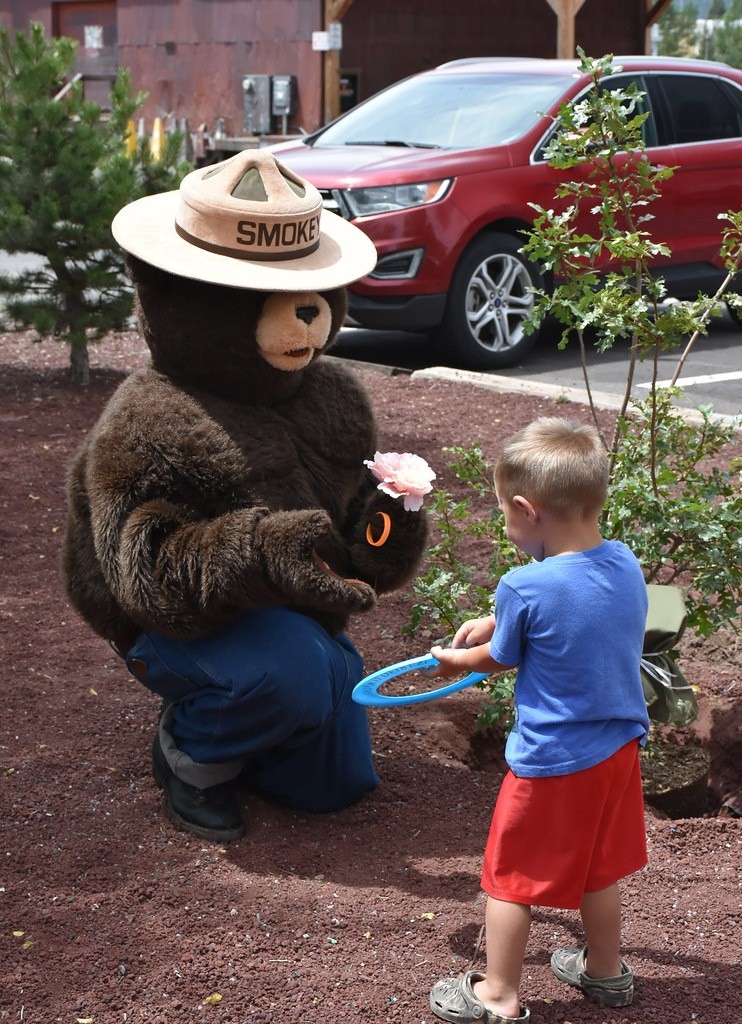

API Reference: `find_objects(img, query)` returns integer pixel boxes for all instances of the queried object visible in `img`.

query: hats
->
[111,149,377,291]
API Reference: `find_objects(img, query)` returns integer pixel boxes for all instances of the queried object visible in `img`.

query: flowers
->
[363,450,437,514]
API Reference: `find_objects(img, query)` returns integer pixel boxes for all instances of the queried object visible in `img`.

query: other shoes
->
[550,944,633,1007]
[430,971,531,1024]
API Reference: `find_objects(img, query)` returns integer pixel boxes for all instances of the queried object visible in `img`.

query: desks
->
[212,136,260,152]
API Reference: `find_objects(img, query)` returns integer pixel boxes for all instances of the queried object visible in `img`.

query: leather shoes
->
[151,733,245,840]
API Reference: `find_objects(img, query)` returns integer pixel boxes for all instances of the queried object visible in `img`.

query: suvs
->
[258,51,742,369]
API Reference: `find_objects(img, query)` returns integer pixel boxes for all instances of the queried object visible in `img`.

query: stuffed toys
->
[58,149,432,834]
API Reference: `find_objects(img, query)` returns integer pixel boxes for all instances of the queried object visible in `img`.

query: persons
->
[427,418,646,1024]
